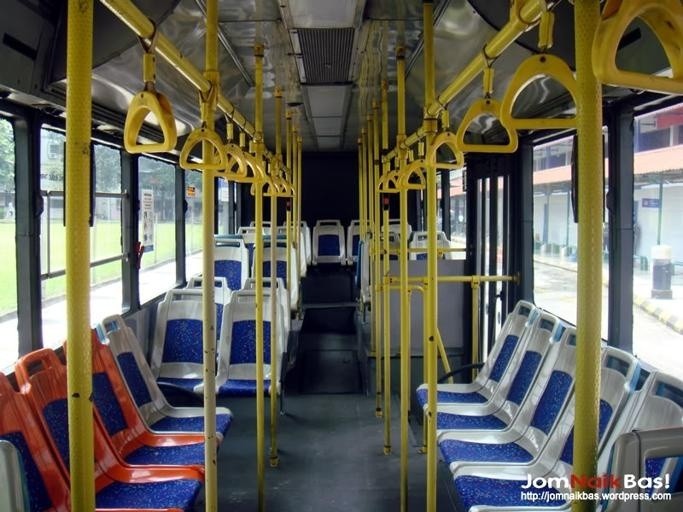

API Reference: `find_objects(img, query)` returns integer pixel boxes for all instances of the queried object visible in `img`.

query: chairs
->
[14,347,205,512]
[0,372,71,512]
[437,327,607,476]
[96,313,234,446]
[565,369,682,512]
[434,309,560,440]
[62,327,221,475]
[453,347,641,510]
[415,299,537,412]
